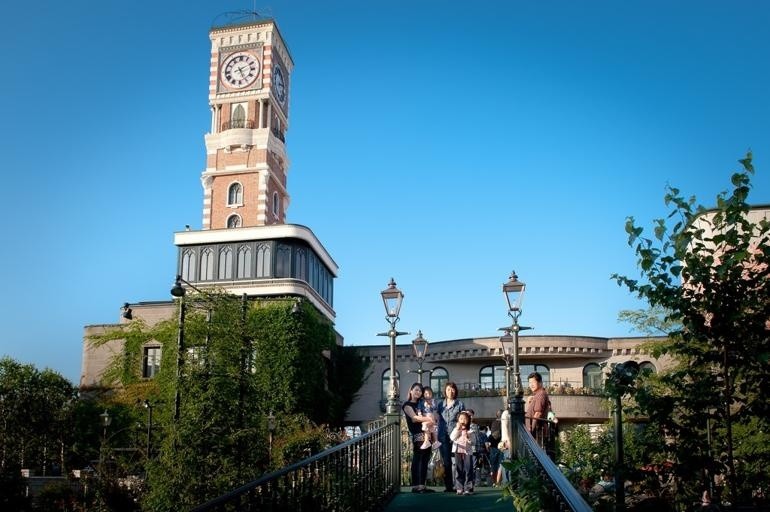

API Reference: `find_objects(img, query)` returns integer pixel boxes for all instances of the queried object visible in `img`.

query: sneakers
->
[421,441,431,449]
[412,486,473,495]
[432,441,442,449]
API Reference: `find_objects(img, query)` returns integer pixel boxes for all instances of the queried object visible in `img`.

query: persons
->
[401,372,559,497]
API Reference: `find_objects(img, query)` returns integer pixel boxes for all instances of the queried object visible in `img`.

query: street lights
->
[500,266,534,395]
[373,273,411,413]
[121,274,252,494]
[97,405,113,480]
[494,328,523,401]
[605,359,648,509]
[404,327,436,394]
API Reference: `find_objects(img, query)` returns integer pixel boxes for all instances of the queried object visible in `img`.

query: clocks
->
[272,65,286,105]
[217,50,261,88]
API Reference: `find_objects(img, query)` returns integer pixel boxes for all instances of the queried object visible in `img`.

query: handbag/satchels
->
[498,441,507,452]
[427,460,446,480]
